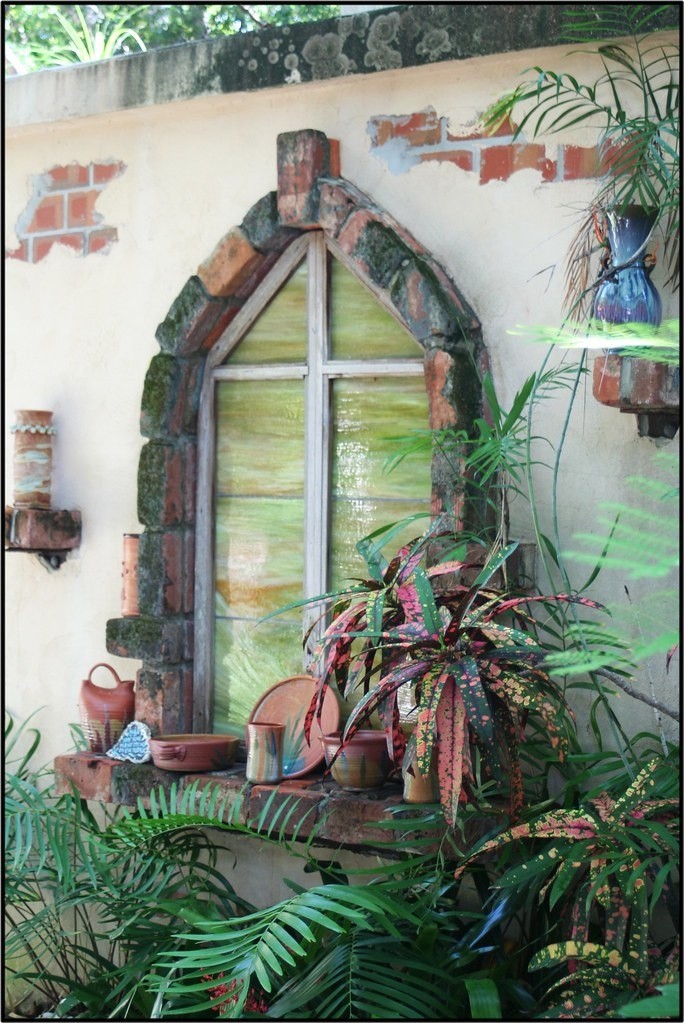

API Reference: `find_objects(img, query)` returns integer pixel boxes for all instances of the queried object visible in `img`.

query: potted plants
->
[254,508,614,826]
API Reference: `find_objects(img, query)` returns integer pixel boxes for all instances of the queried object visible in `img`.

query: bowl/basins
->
[319,729,391,791]
[149,734,238,772]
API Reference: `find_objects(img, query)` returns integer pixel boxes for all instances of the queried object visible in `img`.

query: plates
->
[244,674,340,778]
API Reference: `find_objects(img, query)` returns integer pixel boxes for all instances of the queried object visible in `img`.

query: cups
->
[247,723,286,784]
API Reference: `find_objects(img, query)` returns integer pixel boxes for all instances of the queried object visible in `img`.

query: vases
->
[592,203,663,352]
[320,730,397,792]
[12,409,55,509]
